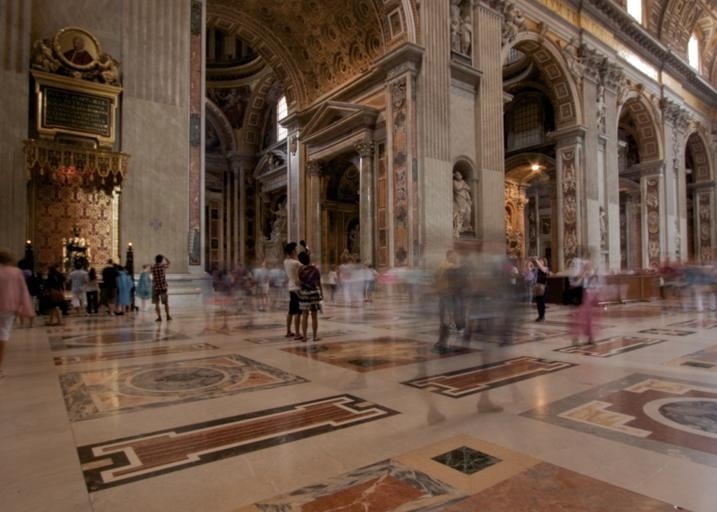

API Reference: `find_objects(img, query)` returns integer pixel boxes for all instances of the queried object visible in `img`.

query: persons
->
[0,238,717,425]
[63,35,94,65]
[452,171,474,230]
[269,203,287,245]
[448,13,474,55]
[505,2,526,42]
